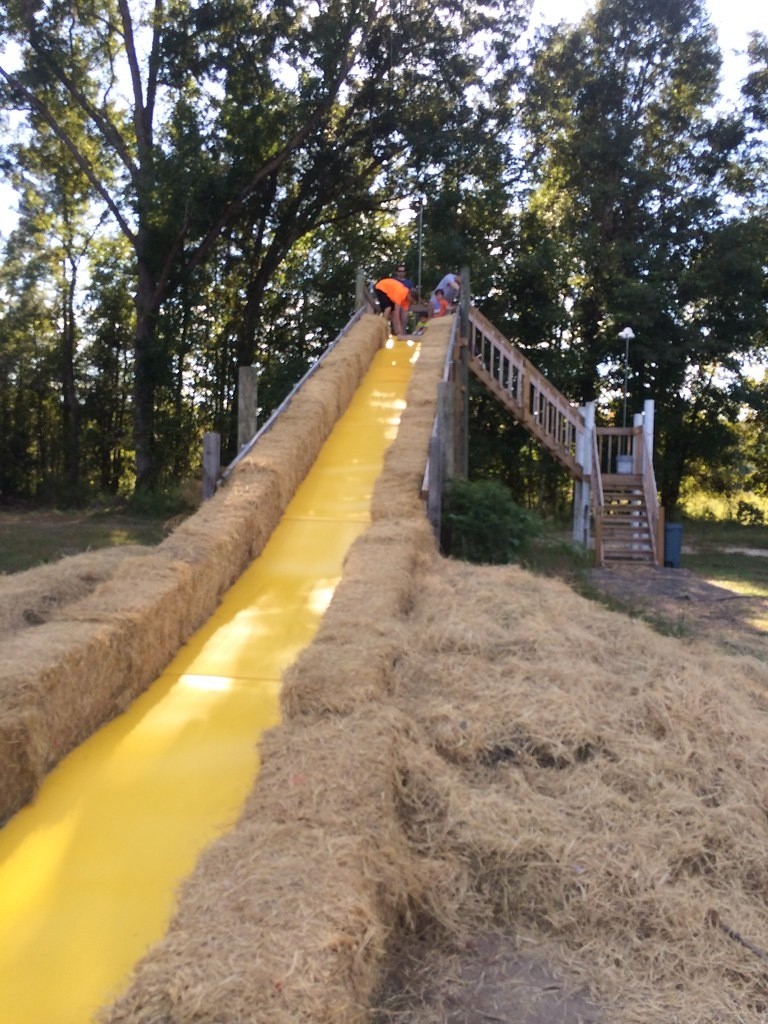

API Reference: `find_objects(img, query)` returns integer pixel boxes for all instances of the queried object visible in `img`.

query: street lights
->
[618,326,637,454]
[410,197,423,303]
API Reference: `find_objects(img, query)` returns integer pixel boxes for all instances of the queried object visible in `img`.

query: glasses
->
[398,270,406,272]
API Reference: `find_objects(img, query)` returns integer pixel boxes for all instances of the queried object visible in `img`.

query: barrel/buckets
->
[617,456,632,474]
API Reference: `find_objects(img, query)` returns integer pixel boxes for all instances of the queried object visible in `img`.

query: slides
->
[0,330,458,1024]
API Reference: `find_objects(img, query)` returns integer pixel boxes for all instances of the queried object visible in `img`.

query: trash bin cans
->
[665,523,683,568]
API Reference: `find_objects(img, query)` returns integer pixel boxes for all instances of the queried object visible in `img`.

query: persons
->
[415,311,431,336]
[431,288,458,318]
[388,264,425,336]
[429,268,463,305]
[373,277,419,342]
